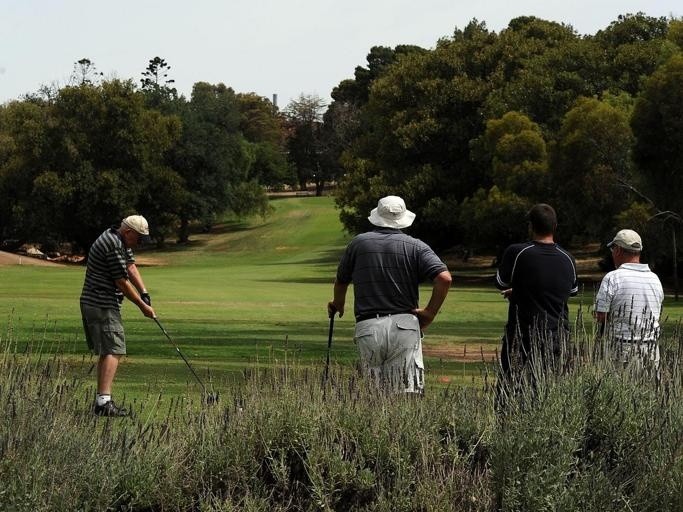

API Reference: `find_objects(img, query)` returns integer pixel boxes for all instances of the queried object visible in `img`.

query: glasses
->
[610,246,618,253]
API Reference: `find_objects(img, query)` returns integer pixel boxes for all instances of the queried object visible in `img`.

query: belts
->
[616,338,654,344]
[355,310,416,323]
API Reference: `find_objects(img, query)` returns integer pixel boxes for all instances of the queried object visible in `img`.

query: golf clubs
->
[153,318,219,404]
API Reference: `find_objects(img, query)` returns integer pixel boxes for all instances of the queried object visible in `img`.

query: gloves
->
[141,292,151,307]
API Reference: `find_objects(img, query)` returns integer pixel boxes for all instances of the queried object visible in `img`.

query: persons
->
[495,203,581,390]
[592,227,665,375]
[326,195,452,397]
[78,213,157,417]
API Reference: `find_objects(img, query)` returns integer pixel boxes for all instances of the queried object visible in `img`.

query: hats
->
[367,195,418,230]
[122,214,151,237]
[607,229,643,252]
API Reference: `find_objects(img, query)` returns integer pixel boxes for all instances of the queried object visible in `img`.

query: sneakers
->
[94,400,131,417]
